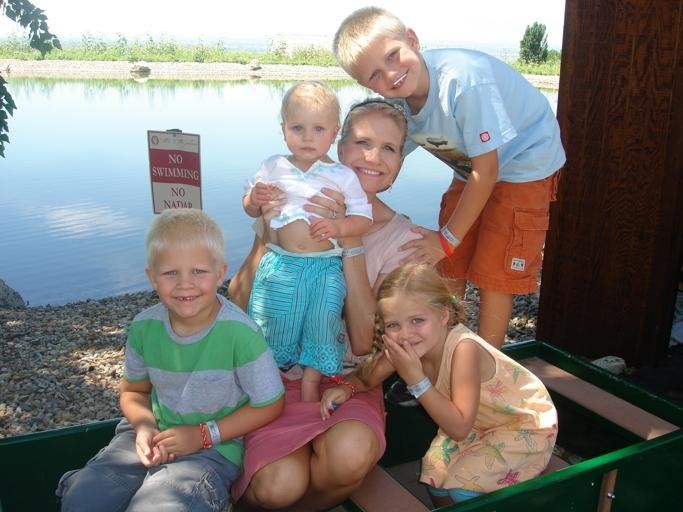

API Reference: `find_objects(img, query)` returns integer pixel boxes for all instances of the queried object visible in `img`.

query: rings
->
[331,211,337,219]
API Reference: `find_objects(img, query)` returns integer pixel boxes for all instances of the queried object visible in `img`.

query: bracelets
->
[342,245,366,258]
[205,419,222,446]
[330,375,356,399]
[437,231,453,257]
[439,225,462,248]
[198,422,212,450]
[404,375,433,399]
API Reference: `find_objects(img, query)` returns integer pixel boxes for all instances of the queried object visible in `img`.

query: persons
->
[236,78,376,404]
[330,6,569,354]
[51,204,288,511]
[317,262,561,507]
[223,94,440,510]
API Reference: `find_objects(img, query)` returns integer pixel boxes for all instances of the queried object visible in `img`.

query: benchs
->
[516,355,681,443]
[538,453,571,474]
[348,462,431,511]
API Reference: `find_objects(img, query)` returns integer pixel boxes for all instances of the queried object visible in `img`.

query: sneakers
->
[385,376,421,406]
